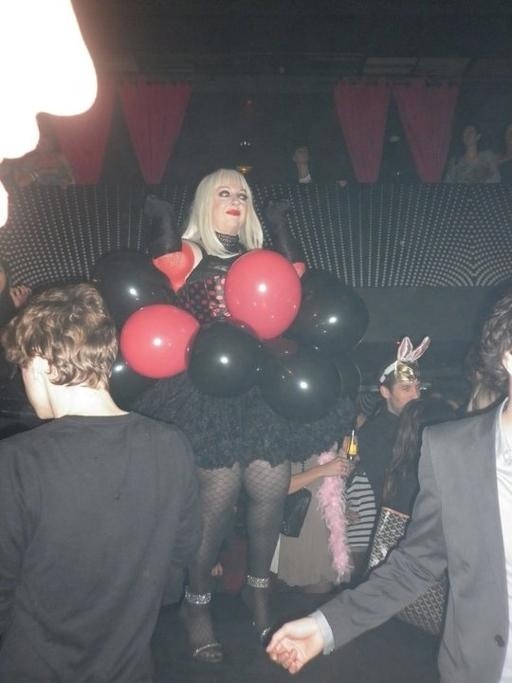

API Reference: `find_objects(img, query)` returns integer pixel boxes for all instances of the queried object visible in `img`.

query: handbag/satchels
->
[365,507,448,632]
[278,487,312,537]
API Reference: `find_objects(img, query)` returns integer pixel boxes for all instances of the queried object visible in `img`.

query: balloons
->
[93,246,368,418]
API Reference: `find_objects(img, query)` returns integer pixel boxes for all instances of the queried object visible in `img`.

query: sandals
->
[245,575,271,637]
[179,588,223,663]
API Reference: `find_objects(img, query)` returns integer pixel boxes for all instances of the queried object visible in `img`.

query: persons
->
[265,299,511,683]
[0,264,192,683]
[149,170,307,666]
